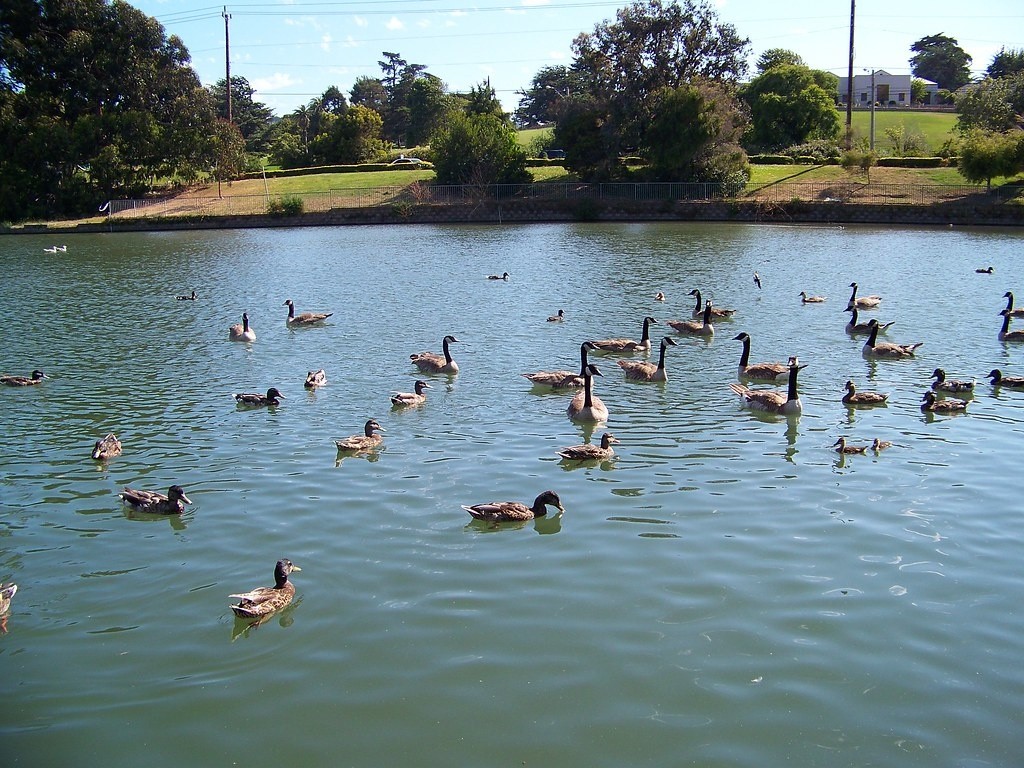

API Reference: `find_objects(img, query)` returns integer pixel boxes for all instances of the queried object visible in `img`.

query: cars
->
[391,155,434,168]
[538,148,568,159]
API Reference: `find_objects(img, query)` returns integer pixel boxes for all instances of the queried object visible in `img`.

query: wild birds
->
[281,299,334,328]
[461,490,566,521]
[91,434,122,460]
[304,369,325,388]
[488,272,1024,453]
[566,363,609,422]
[226,558,302,617]
[229,313,257,342]
[976,266,995,275]
[0,582,18,633]
[555,433,620,460]
[334,420,388,451]
[118,486,193,516]
[390,381,434,406]
[43,245,67,252]
[0,369,49,387]
[410,335,461,375]
[173,291,197,300]
[232,388,286,407]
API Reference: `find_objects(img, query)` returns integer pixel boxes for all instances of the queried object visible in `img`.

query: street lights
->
[863,68,883,151]
